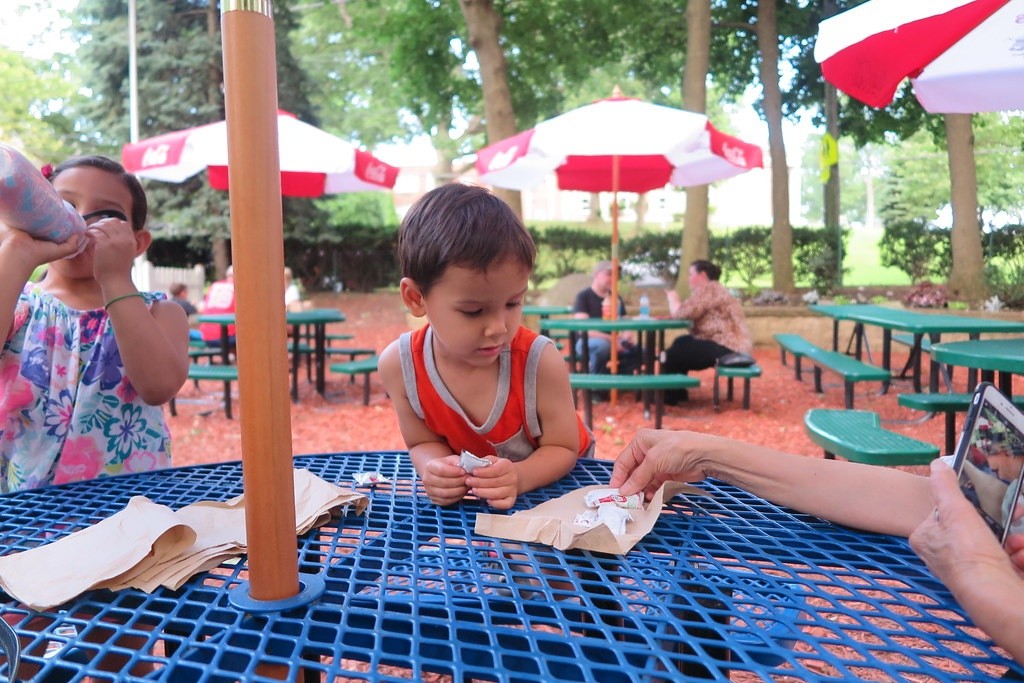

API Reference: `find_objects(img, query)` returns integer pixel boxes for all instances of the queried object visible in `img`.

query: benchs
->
[546,335,764,433]
[179,328,387,415]
[803,406,940,478]
[775,328,1024,455]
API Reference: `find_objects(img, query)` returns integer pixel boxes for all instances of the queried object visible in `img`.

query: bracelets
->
[101,292,150,309]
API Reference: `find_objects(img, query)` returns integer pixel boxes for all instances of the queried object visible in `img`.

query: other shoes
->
[664,389,690,407]
[590,388,606,405]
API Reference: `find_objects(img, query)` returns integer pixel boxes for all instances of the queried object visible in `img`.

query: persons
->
[607,429,1024,673]
[574,259,646,405]
[646,259,751,406]
[377,184,597,640]
[0,150,185,683]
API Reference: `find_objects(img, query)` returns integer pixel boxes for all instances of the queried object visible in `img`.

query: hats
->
[593,261,624,277]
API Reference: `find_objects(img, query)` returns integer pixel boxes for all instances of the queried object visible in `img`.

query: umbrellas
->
[121,109,399,196]
[452,83,764,412]
[814,0,1024,117]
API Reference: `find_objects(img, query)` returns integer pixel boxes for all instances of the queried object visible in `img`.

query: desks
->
[0,451,1024,682]
[190,308,347,416]
[519,305,695,416]
[809,303,1024,452]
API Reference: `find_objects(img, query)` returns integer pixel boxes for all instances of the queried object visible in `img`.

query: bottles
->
[601,294,621,320]
[0,143,92,259]
[638,295,650,319]
